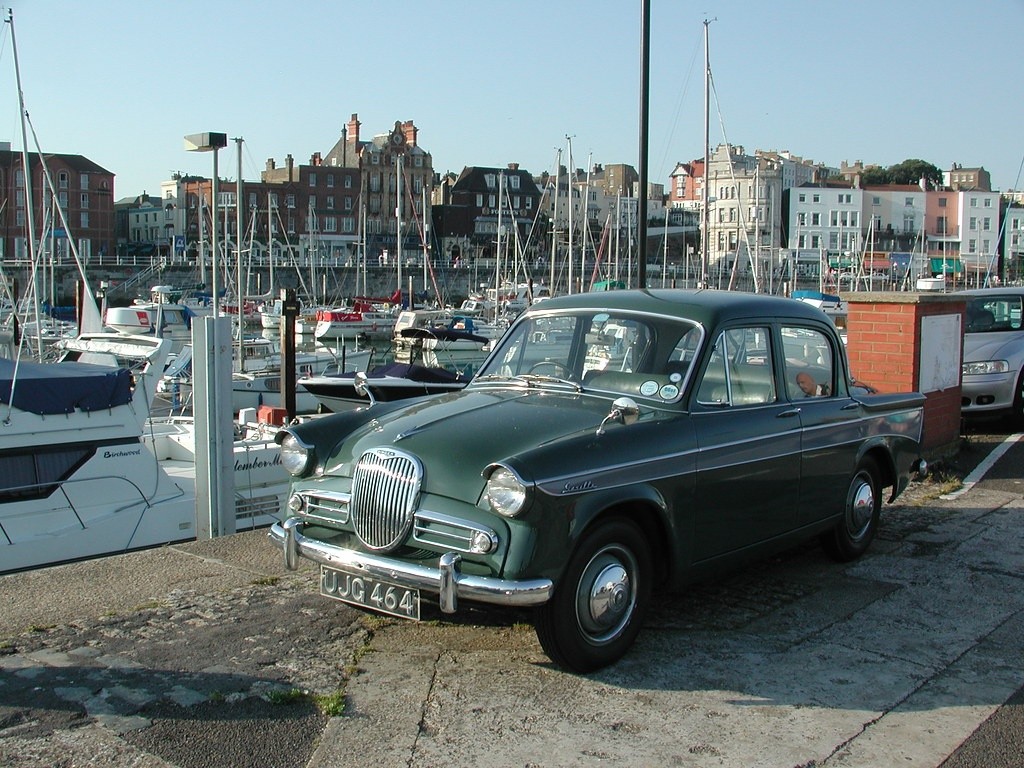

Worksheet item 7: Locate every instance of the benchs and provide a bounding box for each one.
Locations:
[583,358,833,406]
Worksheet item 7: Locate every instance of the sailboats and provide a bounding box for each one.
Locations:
[106,19,961,414]
[1,7,296,573]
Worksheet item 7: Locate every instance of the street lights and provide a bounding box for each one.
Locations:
[185,131,227,538]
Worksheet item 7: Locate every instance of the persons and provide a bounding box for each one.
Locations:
[796,372,831,398]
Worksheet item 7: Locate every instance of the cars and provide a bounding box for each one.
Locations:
[841,271,856,281]
[886,275,902,282]
[265,291,927,677]
[946,287,1024,422]
[861,273,888,282]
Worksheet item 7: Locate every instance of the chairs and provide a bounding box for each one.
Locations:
[971,309,996,328]
